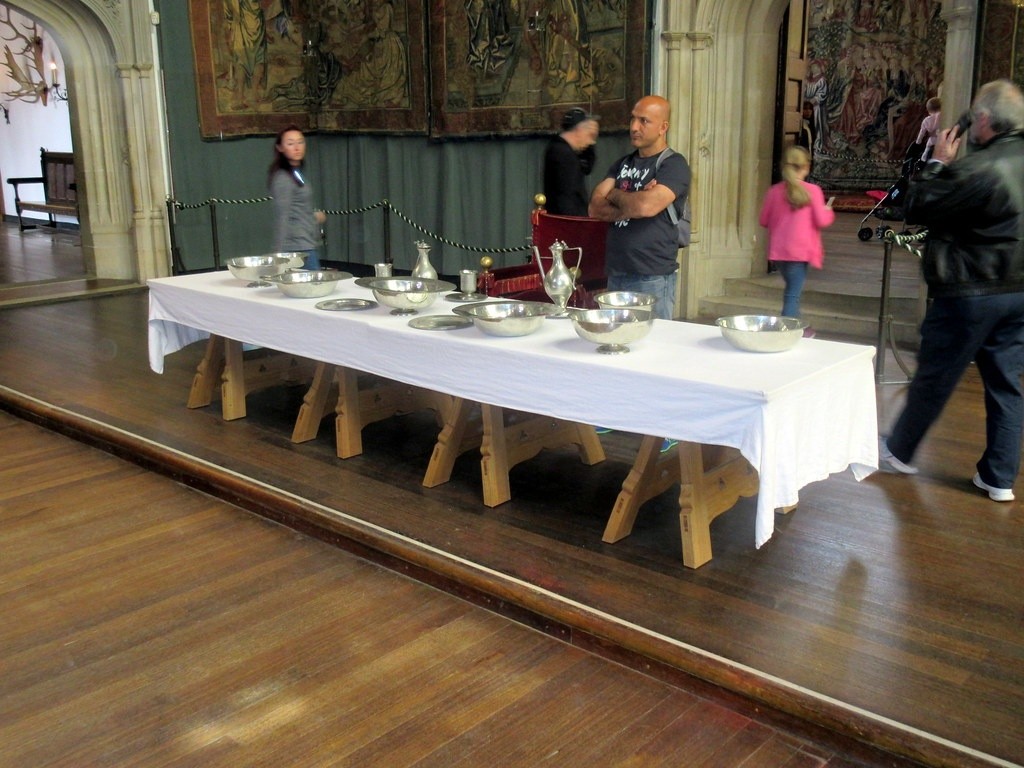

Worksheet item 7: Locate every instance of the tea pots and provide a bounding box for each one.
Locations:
[533,237,582,318]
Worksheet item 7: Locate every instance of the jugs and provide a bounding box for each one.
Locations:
[411,240,439,281]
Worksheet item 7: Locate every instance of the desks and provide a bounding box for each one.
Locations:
[147,270,878,570]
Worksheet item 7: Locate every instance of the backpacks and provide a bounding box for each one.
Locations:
[621,148,693,249]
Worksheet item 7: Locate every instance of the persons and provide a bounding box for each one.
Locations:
[916,96,941,164]
[543,109,596,215]
[798,101,817,156]
[878,78,1024,501]
[266,128,326,268]
[759,145,835,338]
[588,95,692,319]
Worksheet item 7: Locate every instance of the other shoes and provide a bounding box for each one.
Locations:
[802,328,817,339]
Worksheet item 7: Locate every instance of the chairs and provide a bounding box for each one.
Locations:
[6,148,81,238]
[476,194,608,310]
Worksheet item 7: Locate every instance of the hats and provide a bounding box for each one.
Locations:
[560,107,601,131]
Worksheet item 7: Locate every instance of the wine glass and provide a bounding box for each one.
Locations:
[459,269,479,301]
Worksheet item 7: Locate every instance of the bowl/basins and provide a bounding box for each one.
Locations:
[355,276,458,317]
[452,300,564,336]
[593,291,657,316]
[264,252,308,269]
[265,270,352,298]
[224,256,291,287]
[714,314,811,354]
[567,309,656,356]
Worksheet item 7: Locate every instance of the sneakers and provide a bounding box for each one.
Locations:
[659,437,679,452]
[878,437,917,474]
[594,426,614,434]
[973,471,1015,502]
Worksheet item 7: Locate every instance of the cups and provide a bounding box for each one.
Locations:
[374,263,392,278]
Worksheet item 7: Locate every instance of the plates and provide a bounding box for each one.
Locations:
[445,292,488,304]
[315,299,377,313]
[407,314,475,330]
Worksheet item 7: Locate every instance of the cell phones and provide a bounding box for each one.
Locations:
[946,110,974,141]
